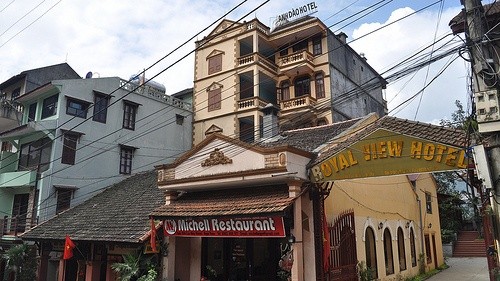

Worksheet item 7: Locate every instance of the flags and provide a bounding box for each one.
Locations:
[150,216,157,253]
[63,234,76,259]
[321,211,332,272]
